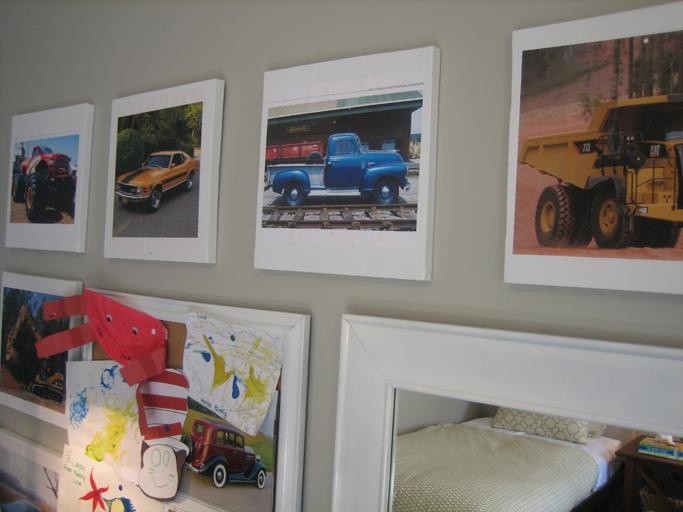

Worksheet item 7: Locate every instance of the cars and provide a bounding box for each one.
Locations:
[116,147,197,210]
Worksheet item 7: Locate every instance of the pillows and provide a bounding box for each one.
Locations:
[489,406,606,446]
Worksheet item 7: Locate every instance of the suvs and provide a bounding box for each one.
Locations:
[13,144,81,223]
[182,418,267,492]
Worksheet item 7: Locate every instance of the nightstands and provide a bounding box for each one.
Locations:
[614,435,683,512]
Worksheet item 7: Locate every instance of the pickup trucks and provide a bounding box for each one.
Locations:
[264,130,410,209]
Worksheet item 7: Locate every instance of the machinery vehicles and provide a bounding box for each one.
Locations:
[519,92,681,248]
[2,303,65,403]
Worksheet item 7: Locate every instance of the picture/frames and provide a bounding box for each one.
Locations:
[330,312,683,512]
[1,428,83,511]
[80,288,312,512]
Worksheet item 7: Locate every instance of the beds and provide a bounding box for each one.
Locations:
[391,402,641,512]
